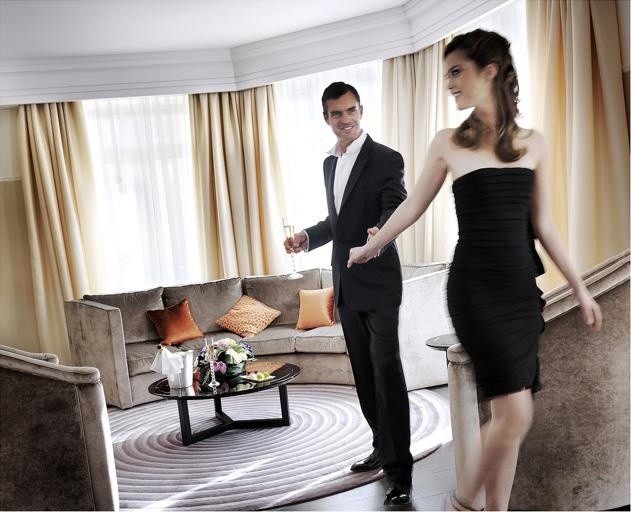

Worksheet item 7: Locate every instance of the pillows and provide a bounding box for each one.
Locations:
[141,295,205,349]
[216,293,285,338]
[292,281,337,333]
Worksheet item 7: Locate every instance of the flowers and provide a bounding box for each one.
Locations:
[192,338,255,386]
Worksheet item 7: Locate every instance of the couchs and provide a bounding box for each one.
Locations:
[61,257,450,408]
[0,345,121,509]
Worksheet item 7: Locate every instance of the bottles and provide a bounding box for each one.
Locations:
[155,344,164,352]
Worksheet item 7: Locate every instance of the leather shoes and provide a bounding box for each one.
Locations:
[351,448,383,472]
[383,481,412,508]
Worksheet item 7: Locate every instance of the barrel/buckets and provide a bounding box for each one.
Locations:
[165,350,195,390]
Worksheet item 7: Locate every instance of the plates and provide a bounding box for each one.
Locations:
[240,372,276,382]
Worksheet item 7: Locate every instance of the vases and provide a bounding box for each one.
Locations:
[214,359,247,379]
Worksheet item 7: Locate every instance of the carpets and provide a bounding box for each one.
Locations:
[105,385,450,509]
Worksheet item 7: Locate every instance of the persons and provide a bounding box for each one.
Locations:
[282,83,415,512]
[348,29,606,512]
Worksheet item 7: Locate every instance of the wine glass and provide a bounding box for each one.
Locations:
[205,337,220,387]
[282,215,305,281]
